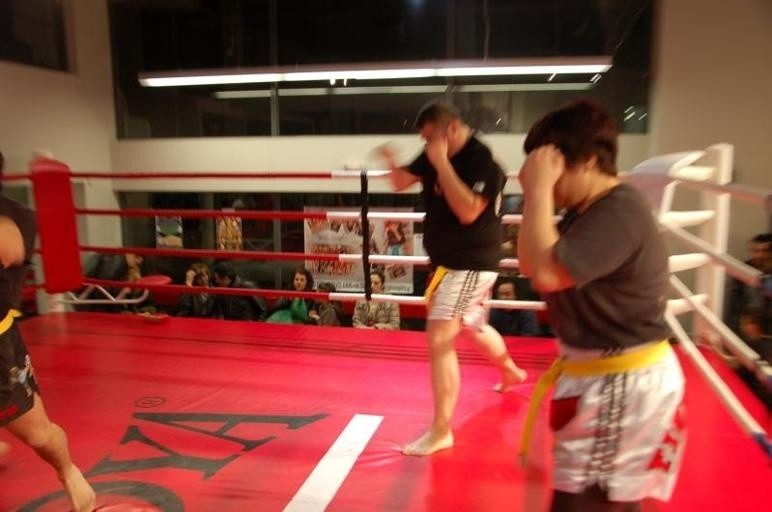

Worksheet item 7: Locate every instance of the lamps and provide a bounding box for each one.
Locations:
[135,4,615,93]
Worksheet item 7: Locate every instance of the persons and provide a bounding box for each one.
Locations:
[0,151,97,512]
[493,282,523,330]
[352,272,401,331]
[517,99,687,512]
[726,232,771,410]
[379,101,528,457]
[175,262,342,326]
